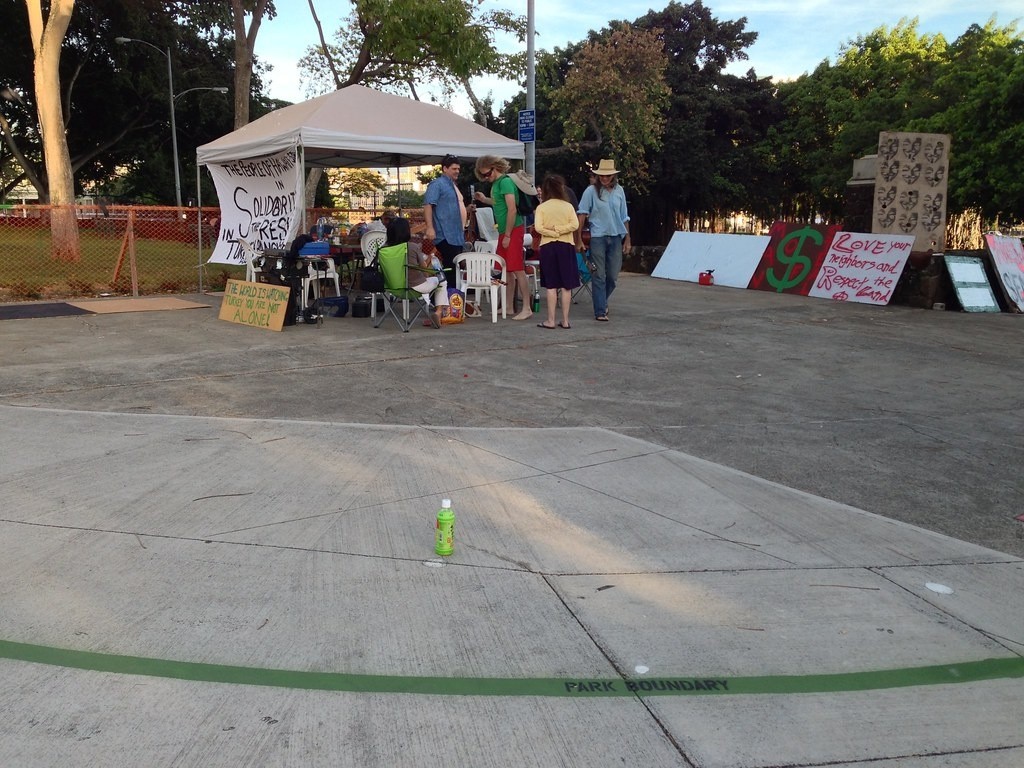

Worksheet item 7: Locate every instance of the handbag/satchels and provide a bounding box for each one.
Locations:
[361,252,384,293]
[440,287,465,323]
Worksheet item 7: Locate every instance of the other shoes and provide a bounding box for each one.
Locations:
[431,314,442,327]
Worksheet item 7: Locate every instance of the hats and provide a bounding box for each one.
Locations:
[507,170,538,196]
[592,159,621,175]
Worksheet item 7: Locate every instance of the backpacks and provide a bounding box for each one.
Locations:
[499,176,540,215]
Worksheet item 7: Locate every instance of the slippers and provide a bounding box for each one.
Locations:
[558,320,571,329]
[597,307,609,321]
[537,321,555,329]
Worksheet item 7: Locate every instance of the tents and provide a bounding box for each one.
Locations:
[194,80,536,296]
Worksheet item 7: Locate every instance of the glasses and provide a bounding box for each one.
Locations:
[481,167,494,177]
[446,155,457,162]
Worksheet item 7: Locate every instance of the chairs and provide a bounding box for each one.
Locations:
[360,229,387,268]
[474,241,495,271]
[570,249,593,304]
[301,257,340,310]
[474,207,499,241]
[453,252,507,324]
[236,237,262,283]
[523,233,540,289]
[373,237,443,332]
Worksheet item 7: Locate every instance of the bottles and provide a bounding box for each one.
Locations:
[530,293,534,312]
[317,215,323,241]
[435,498,455,555]
[430,252,446,282]
[533,291,540,312]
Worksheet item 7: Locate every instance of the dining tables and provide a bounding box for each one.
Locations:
[329,236,365,297]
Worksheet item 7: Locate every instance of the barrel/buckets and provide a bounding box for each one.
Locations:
[699,270,714,285]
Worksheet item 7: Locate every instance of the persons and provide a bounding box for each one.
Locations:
[588,172,598,184]
[377,217,450,327]
[535,184,544,204]
[368,215,388,233]
[473,153,533,320]
[382,211,398,231]
[573,158,631,322]
[533,173,581,329]
